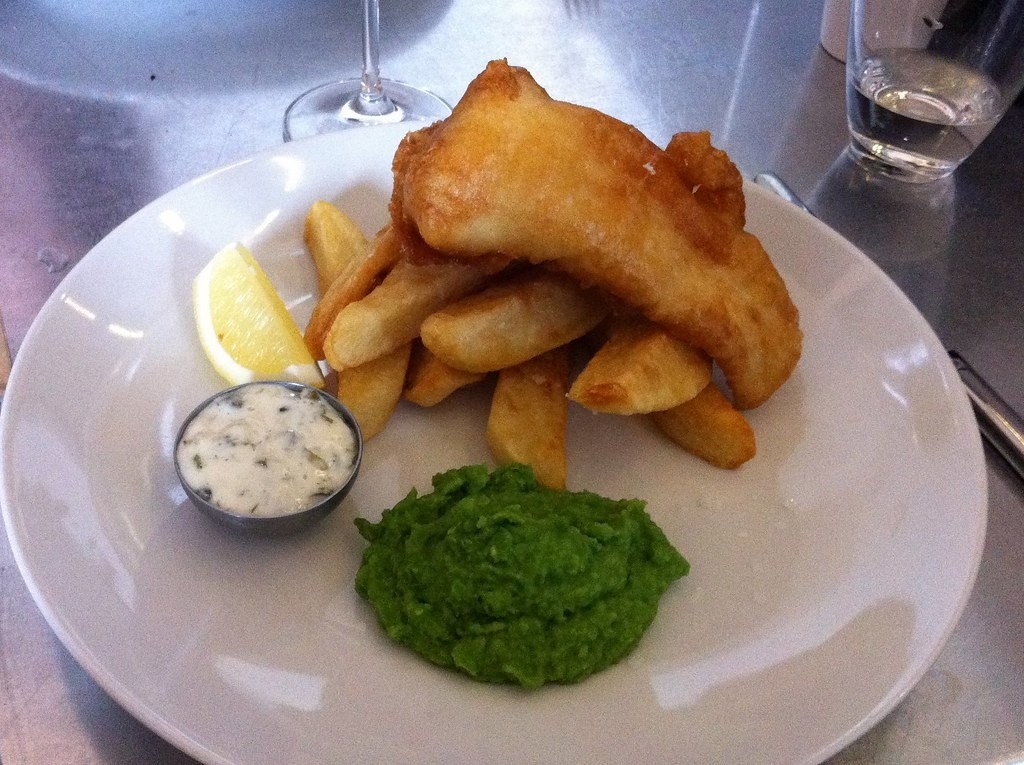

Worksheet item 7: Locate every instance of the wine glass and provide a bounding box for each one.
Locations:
[283,0,454,141]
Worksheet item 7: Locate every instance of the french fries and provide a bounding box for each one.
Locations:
[299,198,756,492]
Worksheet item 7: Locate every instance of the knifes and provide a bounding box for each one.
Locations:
[752,172,1024,481]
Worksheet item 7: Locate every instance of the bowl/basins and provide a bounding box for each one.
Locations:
[173,381,363,536]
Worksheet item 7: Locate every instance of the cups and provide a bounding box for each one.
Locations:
[846,1,1024,184]
[821,1,945,63]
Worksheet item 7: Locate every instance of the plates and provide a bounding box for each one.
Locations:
[0,139,987,764]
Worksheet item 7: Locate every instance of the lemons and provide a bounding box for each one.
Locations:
[193,238,328,393]
